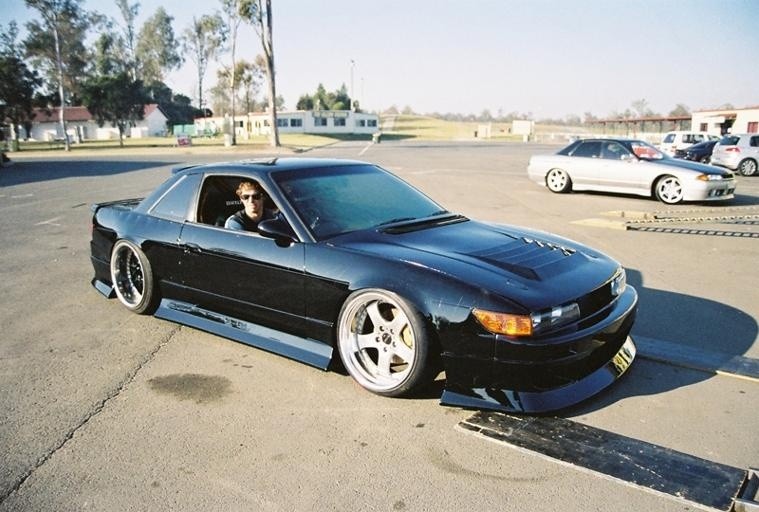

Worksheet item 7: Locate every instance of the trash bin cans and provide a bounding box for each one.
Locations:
[523,135,529,142]
[372,133,382,143]
[7,140,18,152]
[224,133,232,148]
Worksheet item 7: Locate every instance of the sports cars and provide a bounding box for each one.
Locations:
[88,156,638,416]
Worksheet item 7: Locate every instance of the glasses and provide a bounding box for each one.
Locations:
[241,195,262,200]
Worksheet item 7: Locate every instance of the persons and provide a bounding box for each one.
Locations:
[224,178,286,233]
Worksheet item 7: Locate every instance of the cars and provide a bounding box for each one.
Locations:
[527,130,759,205]
